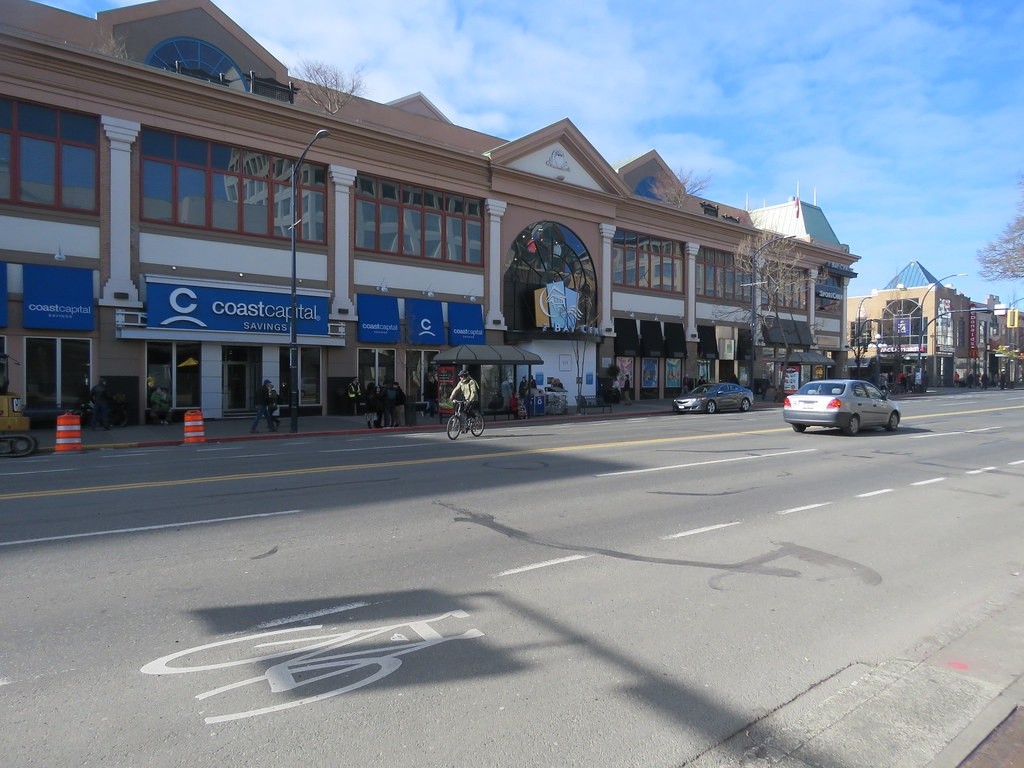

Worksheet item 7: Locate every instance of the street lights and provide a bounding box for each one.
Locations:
[290,130,331,433]
[918,273,969,365]
[750,235,797,388]
[857,296,881,379]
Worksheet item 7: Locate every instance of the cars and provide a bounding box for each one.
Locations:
[783,379,902,436]
[300,376,317,398]
[671,382,754,414]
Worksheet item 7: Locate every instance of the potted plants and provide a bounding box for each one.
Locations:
[608,365,621,383]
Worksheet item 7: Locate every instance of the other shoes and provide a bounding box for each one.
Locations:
[470,418,475,423]
[628,403,632,406]
[624,403,627,405]
[276,420,280,427]
[269,428,278,432]
[460,429,467,434]
[249,429,259,433]
[368,423,401,429]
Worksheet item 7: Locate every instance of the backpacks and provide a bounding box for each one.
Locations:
[399,389,406,405]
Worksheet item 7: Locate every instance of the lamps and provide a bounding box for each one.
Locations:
[0,353,22,364]
[651,313,658,320]
[377,276,389,293]
[707,317,714,324]
[625,310,635,317]
[463,287,478,302]
[422,282,434,297]
[54,242,67,263]
[676,314,683,320]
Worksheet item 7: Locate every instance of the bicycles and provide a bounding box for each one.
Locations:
[70,402,129,429]
[447,400,485,440]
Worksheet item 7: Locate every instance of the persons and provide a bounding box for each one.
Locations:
[879,370,927,393]
[90,377,111,430]
[954,371,1007,390]
[149,386,173,425]
[509,375,537,420]
[623,375,632,405]
[682,373,689,394]
[347,377,407,428]
[698,376,705,385]
[596,371,603,396]
[250,379,279,433]
[422,376,437,417]
[448,370,480,433]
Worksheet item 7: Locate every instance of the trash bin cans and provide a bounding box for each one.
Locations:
[524,389,544,417]
[545,387,569,415]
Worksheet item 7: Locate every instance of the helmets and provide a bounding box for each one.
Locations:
[458,369,469,376]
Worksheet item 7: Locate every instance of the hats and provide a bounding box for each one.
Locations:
[264,380,272,384]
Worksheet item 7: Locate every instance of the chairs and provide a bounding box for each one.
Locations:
[832,388,842,394]
[807,390,817,395]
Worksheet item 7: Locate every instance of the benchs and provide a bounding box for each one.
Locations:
[575,395,612,416]
